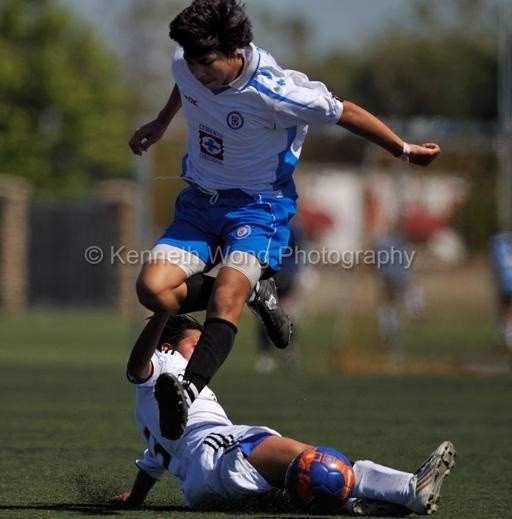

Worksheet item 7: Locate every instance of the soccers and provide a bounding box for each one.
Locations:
[285,447,354,513]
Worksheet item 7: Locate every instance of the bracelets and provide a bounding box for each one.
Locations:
[396,140,410,164]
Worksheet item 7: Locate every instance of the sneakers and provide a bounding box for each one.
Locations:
[417,440,456,514]
[246,278,293,349]
[154,372,188,440]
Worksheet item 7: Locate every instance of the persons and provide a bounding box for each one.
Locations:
[127,1,444,442]
[106,303,458,515]
